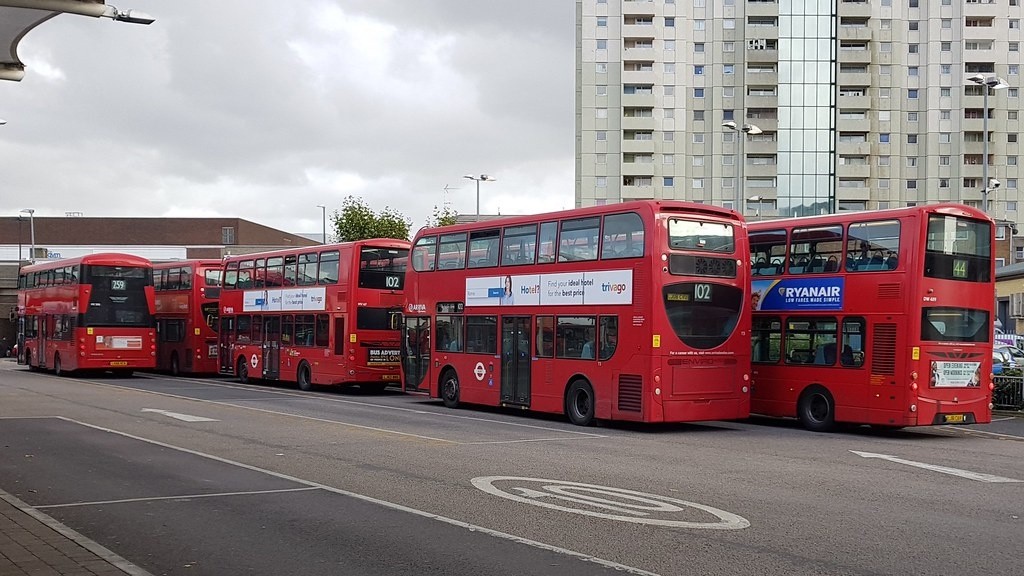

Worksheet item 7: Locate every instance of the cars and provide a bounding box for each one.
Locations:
[992,327,1024,376]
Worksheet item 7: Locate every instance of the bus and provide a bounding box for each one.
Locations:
[399,198,753,429]
[333,229,644,339]
[747,200,996,433]
[152,259,260,379]
[216,234,430,392]
[11,252,158,380]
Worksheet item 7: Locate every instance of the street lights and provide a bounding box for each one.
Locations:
[965,74,1009,214]
[19,208,35,263]
[316,203,326,245]
[721,122,765,218]
[463,174,495,223]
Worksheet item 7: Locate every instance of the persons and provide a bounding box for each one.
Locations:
[500,276,514,305]
[261,290,269,311]
[751,292,761,310]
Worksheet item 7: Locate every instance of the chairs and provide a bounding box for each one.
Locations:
[429,247,641,271]
[244,276,253,289]
[233,278,244,289]
[752,251,896,274]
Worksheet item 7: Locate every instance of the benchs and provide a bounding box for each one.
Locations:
[812,343,854,366]
